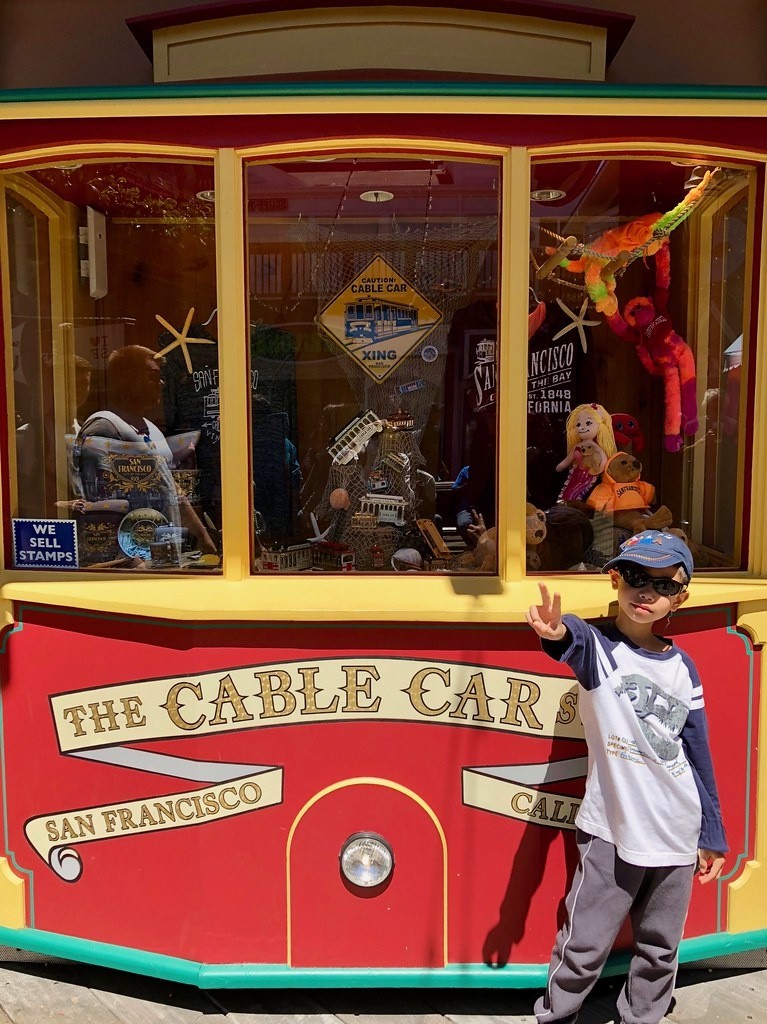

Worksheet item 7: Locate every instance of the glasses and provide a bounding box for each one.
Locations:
[617,565,683,597]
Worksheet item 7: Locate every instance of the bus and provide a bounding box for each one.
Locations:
[326,409,385,466]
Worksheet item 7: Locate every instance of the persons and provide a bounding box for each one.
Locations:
[197,393,307,548]
[526,530,727,1024]
[70,343,221,574]
[17,357,91,518]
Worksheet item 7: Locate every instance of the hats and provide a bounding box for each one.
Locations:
[601,530,694,579]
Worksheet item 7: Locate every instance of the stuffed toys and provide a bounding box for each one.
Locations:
[456,502,546,574]
[582,211,699,452]
[588,451,675,530]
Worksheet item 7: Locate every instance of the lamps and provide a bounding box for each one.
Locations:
[339,832,393,887]
